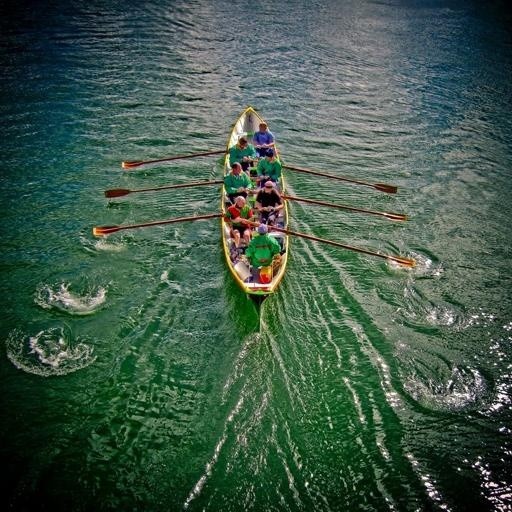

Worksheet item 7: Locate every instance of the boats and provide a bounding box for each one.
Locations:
[221,105,289,295]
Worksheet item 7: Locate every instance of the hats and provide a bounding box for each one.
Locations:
[265,181,273,187]
[258,224,268,234]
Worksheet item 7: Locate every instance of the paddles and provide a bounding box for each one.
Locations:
[93,213,225,237]
[122,151,226,169]
[249,191,408,223]
[105,180,223,198]
[253,160,397,193]
[249,221,415,267]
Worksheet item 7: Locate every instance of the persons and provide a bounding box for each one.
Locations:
[223,195,256,256]
[256,146,282,194]
[222,161,253,208]
[245,221,283,277]
[228,135,256,179]
[254,180,284,232]
[252,121,277,162]
